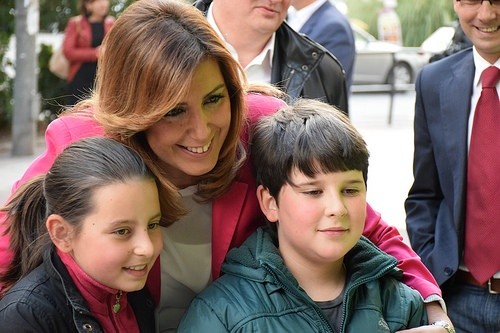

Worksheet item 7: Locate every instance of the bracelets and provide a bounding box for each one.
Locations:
[431,321,456,333]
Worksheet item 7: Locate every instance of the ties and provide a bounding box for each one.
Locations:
[461,65,500,285]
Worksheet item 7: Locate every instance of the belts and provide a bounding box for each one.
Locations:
[456,267,500,294]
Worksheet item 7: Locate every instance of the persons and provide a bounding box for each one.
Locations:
[404,0,500,333]
[290,0,356,104]
[189,0,349,122]
[177,99,429,332]
[0,138,164,333]
[62,0,117,105]
[376,0,403,48]
[0,0,456,332]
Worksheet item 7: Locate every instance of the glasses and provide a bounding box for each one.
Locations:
[455,0,500,8]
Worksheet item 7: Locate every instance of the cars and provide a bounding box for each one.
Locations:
[421,26,456,55]
[350,23,428,85]
